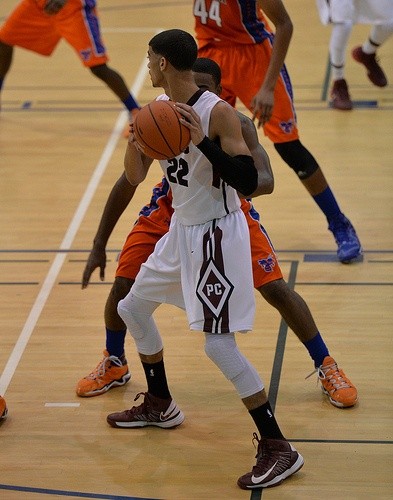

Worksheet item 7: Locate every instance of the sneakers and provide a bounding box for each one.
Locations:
[327,214,362,263]
[125,105,143,137]
[106,392,185,429]
[352,46,389,88]
[330,78,353,110]
[237,432,304,489]
[75,349,130,397]
[319,355,360,408]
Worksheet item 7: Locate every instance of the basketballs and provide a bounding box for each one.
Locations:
[133,100,191,160]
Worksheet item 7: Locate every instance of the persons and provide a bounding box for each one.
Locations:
[0,395,8,427]
[190,0,363,266]
[0,0,142,137]
[105,28,304,490]
[76,57,358,409]
[317,0,393,110]
